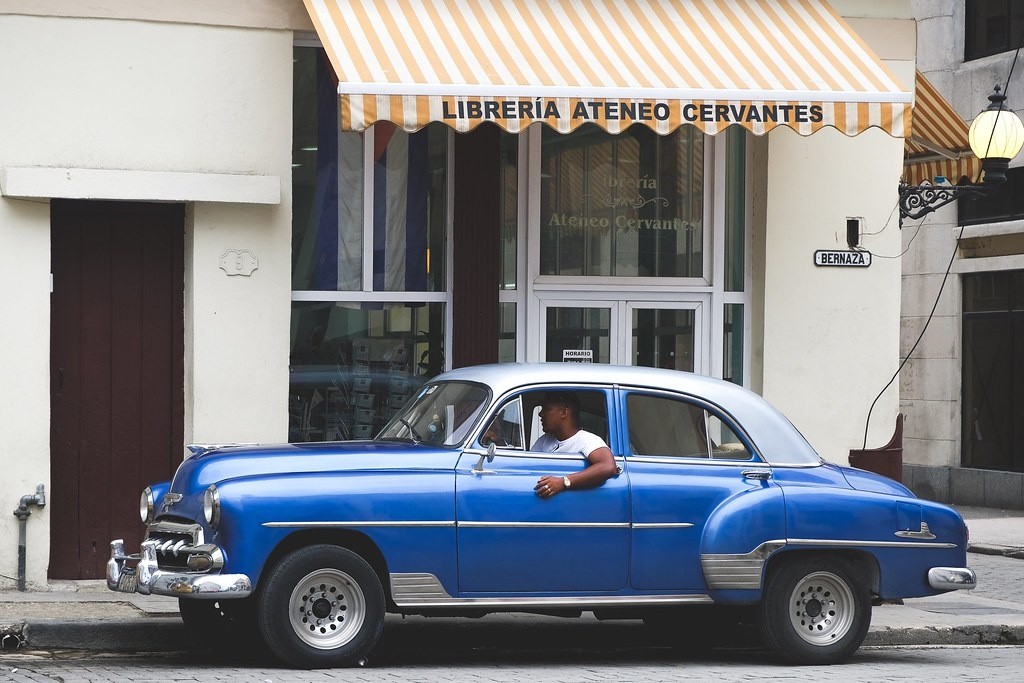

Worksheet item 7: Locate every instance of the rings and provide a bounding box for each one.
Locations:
[545,484,551,493]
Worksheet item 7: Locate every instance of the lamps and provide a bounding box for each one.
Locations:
[897,84,1024,230]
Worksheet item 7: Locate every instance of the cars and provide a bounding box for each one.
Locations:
[107,363,979,669]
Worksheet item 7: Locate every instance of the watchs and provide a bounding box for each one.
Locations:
[563,476,570,489]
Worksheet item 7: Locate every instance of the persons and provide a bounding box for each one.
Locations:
[532,388,618,499]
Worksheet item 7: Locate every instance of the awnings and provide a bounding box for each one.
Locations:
[299,0,912,138]
[905,69,999,188]
